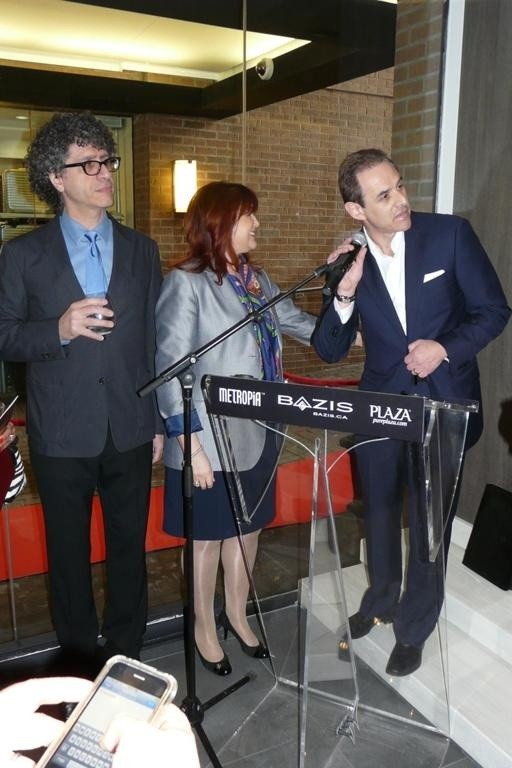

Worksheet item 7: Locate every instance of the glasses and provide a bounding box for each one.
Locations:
[60,157,120,176]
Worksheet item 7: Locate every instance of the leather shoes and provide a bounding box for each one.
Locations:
[349,609,394,640]
[385,642,423,676]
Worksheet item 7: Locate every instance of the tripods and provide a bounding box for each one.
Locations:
[137,265,320,768]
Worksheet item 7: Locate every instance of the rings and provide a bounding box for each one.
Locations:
[414,369,419,375]
[193,480,199,485]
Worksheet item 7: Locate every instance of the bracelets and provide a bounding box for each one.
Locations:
[191,446,202,458]
[335,290,356,303]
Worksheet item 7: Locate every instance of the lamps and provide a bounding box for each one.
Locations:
[172,159,198,214]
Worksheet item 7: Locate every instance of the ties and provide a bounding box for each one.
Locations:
[84,232,106,301]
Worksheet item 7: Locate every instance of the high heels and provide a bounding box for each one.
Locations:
[189,624,232,677]
[219,607,270,658]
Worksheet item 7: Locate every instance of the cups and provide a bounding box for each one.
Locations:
[84,293,116,336]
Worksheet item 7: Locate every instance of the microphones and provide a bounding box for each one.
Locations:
[321,229,367,295]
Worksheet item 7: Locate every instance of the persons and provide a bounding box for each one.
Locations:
[311,150,511,676]
[0,676,202,768]
[0,111,168,681]
[155,183,364,674]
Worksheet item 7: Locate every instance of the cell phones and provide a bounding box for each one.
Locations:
[36,653,176,768]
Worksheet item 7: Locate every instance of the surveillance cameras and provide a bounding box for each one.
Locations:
[256,57,274,81]
